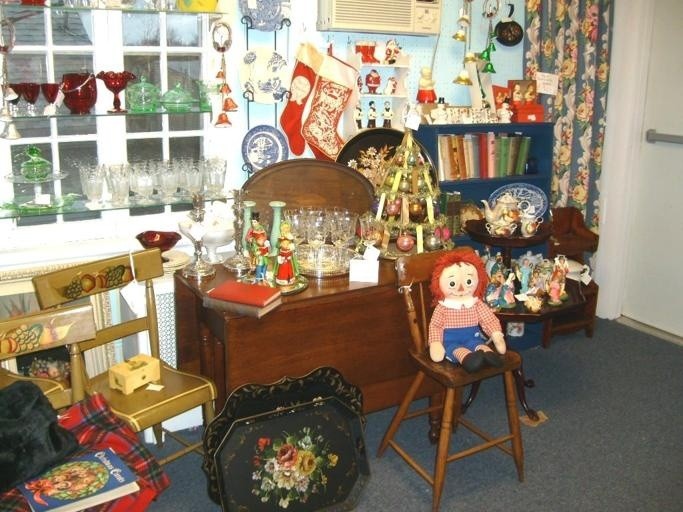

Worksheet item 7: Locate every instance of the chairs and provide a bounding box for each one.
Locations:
[0,302,169,511]
[31,246,218,467]
[379,245,525,512]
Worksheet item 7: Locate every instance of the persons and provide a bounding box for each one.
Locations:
[244,212,297,290]
[421,247,508,371]
[486,253,568,315]
[350,39,540,129]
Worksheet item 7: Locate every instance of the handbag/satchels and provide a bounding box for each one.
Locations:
[0,380,79,491]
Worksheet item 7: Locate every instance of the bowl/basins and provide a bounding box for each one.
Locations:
[158,81,200,113]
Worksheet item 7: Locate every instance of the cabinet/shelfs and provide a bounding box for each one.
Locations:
[342,42,410,137]
[462,208,598,348]
[2,2,234,221]
[419,122,551,351]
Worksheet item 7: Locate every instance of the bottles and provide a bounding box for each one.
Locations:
[520,214,537,237]
[266,200,286,249]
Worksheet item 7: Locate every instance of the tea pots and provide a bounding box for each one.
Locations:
[478,192,521,238]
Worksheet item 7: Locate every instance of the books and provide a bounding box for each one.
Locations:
[18,446,141,511]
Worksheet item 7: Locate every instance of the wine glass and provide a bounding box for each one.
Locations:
[1,81,59,118]
[94,70,137,114]
[283,204,378,273]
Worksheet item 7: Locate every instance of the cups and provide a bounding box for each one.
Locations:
[76,156,228,208]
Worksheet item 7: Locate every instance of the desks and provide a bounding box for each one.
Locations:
[174,259,446,446]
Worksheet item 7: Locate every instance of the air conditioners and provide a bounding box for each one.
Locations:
[317,0,440,38]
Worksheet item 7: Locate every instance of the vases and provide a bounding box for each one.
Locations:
[268,201,287,254]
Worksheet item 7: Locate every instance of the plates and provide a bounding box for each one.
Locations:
[488,182,549,224]
[241,124,290,174]
[237,0,290,33]
[237,48,291,105]
